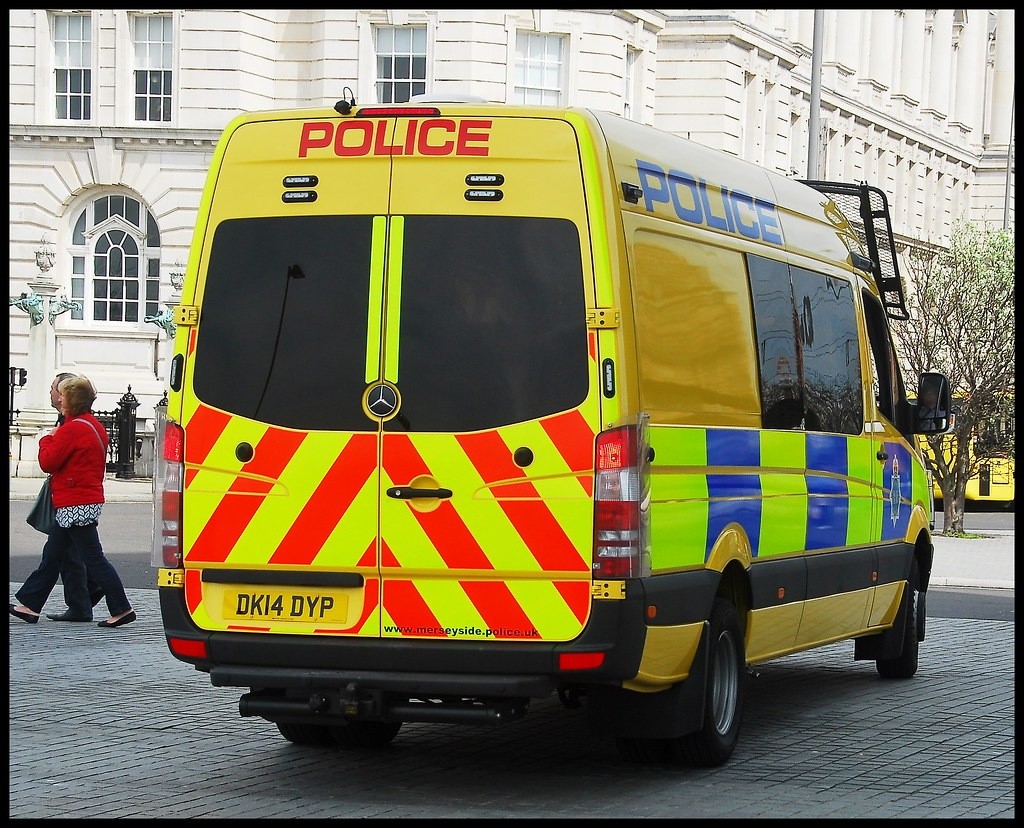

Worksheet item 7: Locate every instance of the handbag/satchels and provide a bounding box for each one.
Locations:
[26,474,57,536]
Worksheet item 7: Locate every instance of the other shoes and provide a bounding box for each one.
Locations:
[9,603,39,624]
[46,609,94,624]
[97,611,137,628]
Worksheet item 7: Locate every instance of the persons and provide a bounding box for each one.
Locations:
[919,387,946,431]
[9,372,136,629]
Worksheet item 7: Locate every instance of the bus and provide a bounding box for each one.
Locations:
[903,383,1017,512]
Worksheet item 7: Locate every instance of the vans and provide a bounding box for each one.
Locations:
[156,86,954,776]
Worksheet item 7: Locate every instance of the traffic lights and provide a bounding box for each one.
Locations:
[19,368,28,387]
